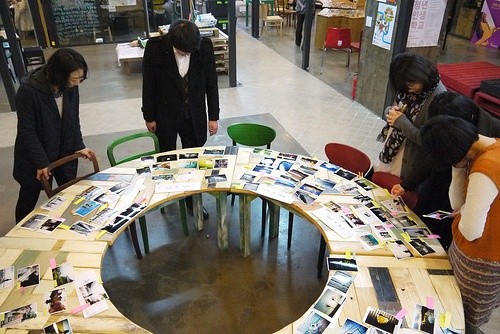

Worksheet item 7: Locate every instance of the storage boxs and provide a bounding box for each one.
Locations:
[23,46,45,72]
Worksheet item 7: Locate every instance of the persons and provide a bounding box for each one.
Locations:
[292,0,315,51]
[17,265,39,288]
[375,53,447,178]
[13,49,95,225]
[391,91,500,334]
[141,20,220,218]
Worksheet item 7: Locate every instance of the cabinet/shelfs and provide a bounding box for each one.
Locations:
[112,13,136,33]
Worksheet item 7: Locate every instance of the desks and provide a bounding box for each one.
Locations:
[314,0,365,49]
[435,60,500,118]
[0,146,466,334]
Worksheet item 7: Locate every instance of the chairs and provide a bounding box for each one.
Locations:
[348,28,363,68]
[261,4,283,38]
[290,143,420,281]
[277,0,297,28]
[108,132,188,255]
[39,152,143,259]
[227,124,277,206]
[320,27,351,75]
